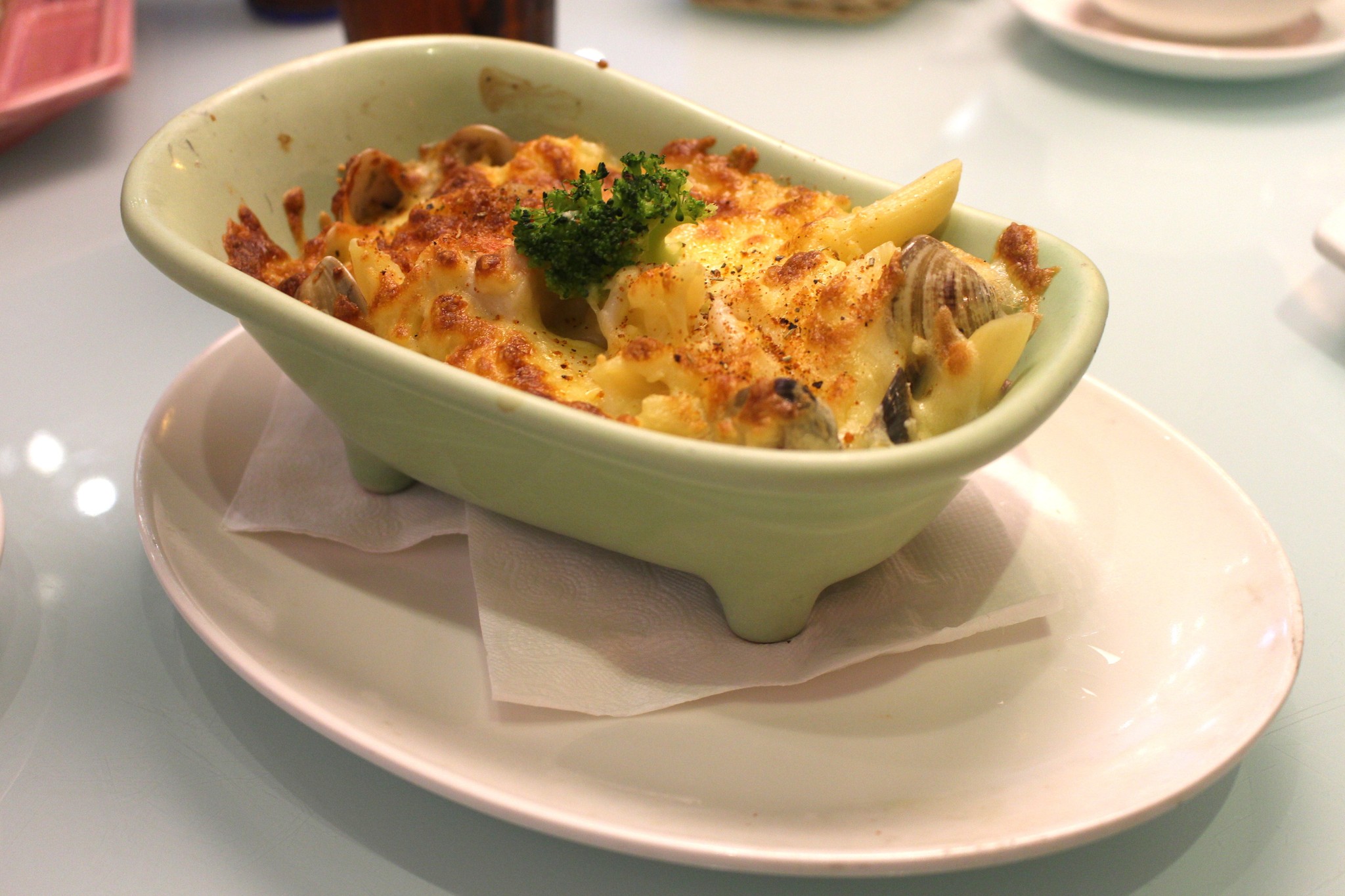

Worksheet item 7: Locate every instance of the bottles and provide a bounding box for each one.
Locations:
[339,0,556,52]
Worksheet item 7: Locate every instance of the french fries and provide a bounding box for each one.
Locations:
[967,313,1034,403]
[812,157,961,256]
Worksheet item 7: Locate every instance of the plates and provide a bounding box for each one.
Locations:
[1016,0,1345,78]
[135,328,1306,877]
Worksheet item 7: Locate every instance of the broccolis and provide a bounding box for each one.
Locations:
[509,147,718,303]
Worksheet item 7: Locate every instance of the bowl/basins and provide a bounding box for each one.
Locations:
[122,33,1107,643]
